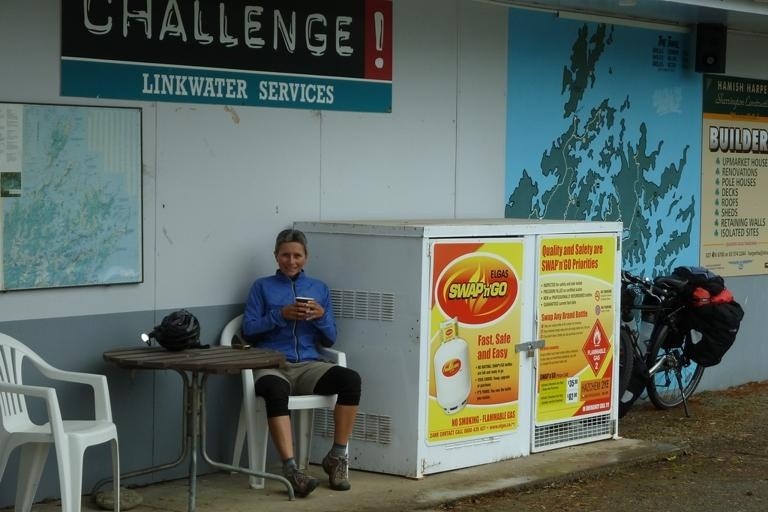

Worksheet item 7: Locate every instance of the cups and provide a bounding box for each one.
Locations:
[294,295,315,305]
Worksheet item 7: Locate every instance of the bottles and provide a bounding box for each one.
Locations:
[432,316,472,414]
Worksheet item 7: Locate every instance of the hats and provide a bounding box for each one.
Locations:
[619,282,641,322]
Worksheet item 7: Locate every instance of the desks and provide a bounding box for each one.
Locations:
[99,342,286,512]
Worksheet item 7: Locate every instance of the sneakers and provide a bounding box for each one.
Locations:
[287,465,320,499]
[321,451,353,492]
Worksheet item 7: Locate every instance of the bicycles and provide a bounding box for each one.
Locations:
[619,269,706,419]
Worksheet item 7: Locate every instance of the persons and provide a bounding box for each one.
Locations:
[241,228,363,496]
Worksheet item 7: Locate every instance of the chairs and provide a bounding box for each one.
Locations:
[219,310,352,491]
[0,330,123,512]
